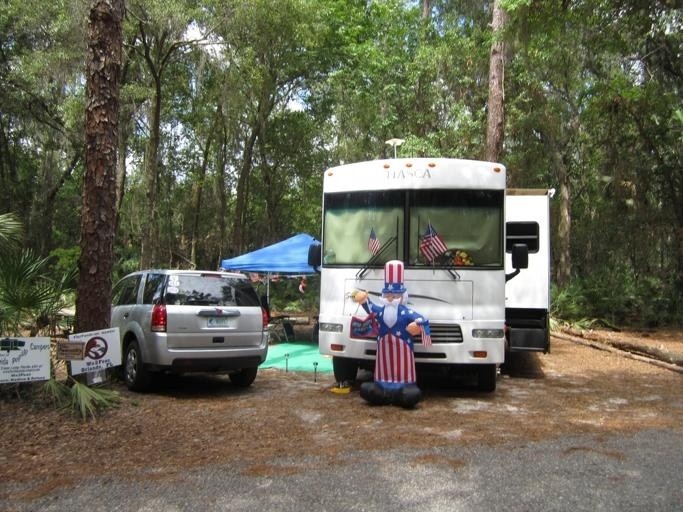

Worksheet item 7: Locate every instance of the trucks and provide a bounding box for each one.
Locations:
[317,157,550,392]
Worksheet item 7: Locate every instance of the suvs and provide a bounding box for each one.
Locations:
[104,269,268,388]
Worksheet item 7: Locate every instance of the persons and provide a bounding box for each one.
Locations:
[351,260,432,410]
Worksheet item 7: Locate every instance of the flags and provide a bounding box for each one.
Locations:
[368,227,380,256]
[420,223,447,263]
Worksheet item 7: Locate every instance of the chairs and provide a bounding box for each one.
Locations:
[260,295,291,342]
